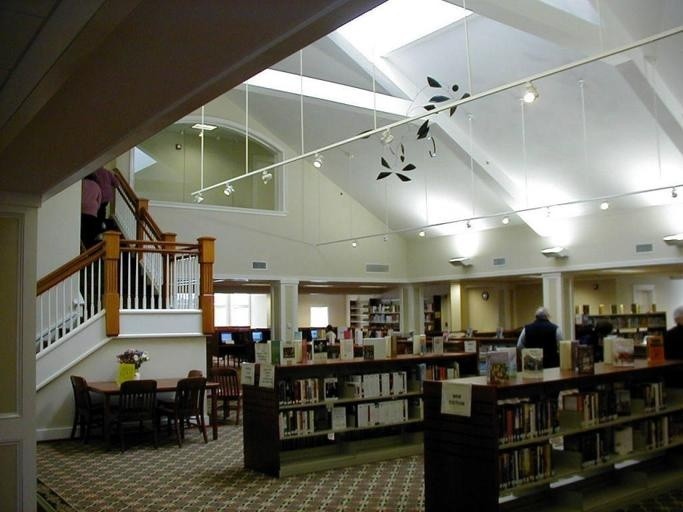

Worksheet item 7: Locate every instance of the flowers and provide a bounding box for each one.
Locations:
[117,347,150,369]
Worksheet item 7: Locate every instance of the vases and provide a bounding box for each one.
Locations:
[118,362,138,383]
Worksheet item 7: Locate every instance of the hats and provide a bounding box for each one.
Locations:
[535,308,551,317]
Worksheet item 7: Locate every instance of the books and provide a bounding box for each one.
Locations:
[255,335,476,439]
[587,314,664,347]
[480,336,665,385]
[371,303,435,331]
[497,381,683,491]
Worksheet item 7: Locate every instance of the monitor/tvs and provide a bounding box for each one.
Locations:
[252,331,264,343]
[311,329,319,339]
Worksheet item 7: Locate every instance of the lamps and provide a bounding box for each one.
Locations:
[311,60,682,251]
[192,124,217,131]
[189,1,679,205]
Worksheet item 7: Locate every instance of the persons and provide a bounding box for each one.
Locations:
[326,325,336,344]
[576,317,613,362]
[81,175,102,238]
[344,327,352,339]
[517,306,563,368]
[375,327,382,338]
[664,306,683,360]
[94,167,118,222]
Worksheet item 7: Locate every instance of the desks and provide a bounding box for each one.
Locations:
[88,379,221,403]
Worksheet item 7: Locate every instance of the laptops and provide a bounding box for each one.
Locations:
[220,331,232,344]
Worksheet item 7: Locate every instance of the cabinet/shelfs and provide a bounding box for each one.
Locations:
[590,314,667,359]
[419,355,683,512]
[349,299,401,338]
[240,348,483,477]
[326,296,397,331]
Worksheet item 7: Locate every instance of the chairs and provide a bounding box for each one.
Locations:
[103,379,160,452]
[210,368,242,424]
[68,375,118,447]
[158,377,209,448]
